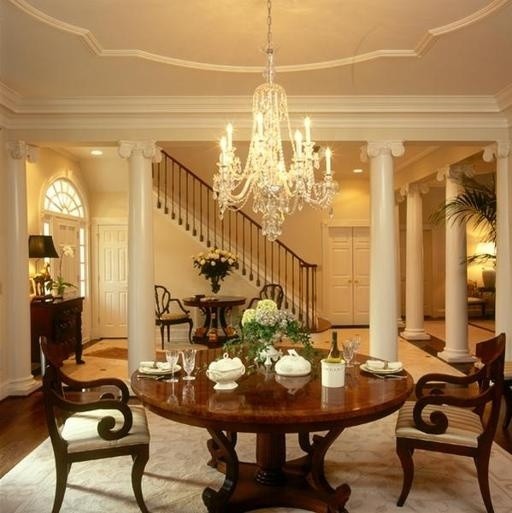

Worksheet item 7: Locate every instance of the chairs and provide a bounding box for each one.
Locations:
[36,334,153,513]
[466,279,489,318]
[393,332,506,511]
[238,284,284,343]
[154,283,193,351]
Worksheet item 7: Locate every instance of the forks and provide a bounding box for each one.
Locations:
[136,373,165,380]
[373,373,408,379]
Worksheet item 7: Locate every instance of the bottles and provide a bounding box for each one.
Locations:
[326,330,341,362]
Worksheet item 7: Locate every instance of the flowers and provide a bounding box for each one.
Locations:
[44,242,78,289]
[219,300,317,339]
[191,248,238,286]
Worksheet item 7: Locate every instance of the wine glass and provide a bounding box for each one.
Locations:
[342,340,354,367]
[351,333,361,364]
[164,348,179,382]
[180,346,196,380]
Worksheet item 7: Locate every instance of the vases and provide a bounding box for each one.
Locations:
[247,330,285,370]
[205,281,224,298]
[51,286,64,298]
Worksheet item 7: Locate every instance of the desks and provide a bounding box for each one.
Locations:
[476,285,496,317]
[182,293,246,349]
[130,345,415,513]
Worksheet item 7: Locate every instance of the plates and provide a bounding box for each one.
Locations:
[137,361,182,374]
[360,363,404,373]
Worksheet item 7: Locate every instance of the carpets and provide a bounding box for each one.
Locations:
[0,401,512,513]
[54,323,469,388]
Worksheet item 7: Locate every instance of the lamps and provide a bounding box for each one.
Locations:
[27,234,59,298]
[475,241,496,288]
[209,2,341,244]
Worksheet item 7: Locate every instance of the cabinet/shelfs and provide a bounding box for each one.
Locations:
[30,295,87,375]
